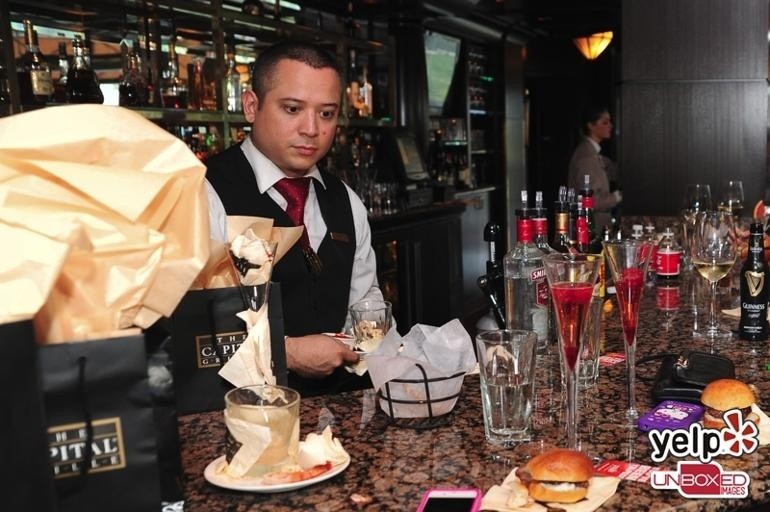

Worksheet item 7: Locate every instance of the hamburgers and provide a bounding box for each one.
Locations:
[515,449,593,504]
[700,378,760,430]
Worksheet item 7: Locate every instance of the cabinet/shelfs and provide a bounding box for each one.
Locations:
[0,1,531,397]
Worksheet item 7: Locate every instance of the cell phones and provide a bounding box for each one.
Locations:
[416,487,483,512]
[637,399,704,433]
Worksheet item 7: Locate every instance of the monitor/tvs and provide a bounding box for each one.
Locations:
[424,30,464,116]
[382,129,432,181]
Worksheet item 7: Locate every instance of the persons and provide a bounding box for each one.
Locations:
[569,107,624,237]
[202,36,396,396]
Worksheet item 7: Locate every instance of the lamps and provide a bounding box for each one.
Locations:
[572,28,614,64]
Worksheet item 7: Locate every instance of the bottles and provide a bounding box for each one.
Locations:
[7,12,399,217]
[502,171,769,361]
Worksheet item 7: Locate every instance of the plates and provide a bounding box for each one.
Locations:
[204,440,352,497]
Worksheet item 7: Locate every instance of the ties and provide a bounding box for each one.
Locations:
[272,177,311,250]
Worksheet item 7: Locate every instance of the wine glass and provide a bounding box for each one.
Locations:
[542,240,654,465]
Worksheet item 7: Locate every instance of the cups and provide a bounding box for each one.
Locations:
[473,329,539,443]
[223,386,302,473]
[343,299,391,347]
